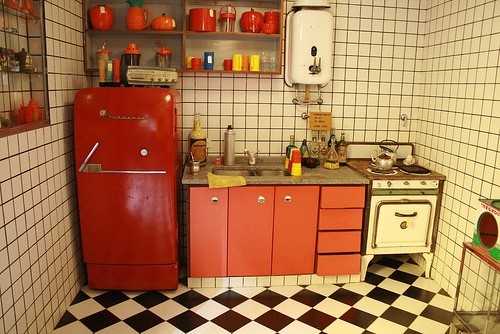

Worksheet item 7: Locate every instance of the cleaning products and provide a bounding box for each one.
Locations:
[284,134,297,169]
[224,125,235,166]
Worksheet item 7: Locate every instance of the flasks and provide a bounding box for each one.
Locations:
[224,124,235,166]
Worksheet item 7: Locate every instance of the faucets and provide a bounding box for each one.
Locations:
[243,149,260,165]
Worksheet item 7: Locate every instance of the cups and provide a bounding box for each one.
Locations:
[224,54,279,71]
[219,4,236,33]
[187,56,201,71]
[0,46,33,72]
[106,57,120,82]
[204,51,214,70]
[190,160,200,175]
[125,6,151,32]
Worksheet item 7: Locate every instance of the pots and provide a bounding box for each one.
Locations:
[240,8,263,33]
[189,7,217,32]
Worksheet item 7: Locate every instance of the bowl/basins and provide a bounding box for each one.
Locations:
[152,13,175,31]
[260,10,280,34]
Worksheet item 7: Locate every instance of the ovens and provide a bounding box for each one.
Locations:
[365,190,439,255]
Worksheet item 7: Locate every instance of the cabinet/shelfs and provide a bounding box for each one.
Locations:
[446,248,500,334]
[188,183,367,276]
[82,0,283,75]
[0,0,50,137]
[364,178,443,279]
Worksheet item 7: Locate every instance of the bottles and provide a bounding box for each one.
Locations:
[300,128,347,171]
[155,46,171,67]
[90,3,115,31]
[288,135,295,145]
[187,112,208,167]
[119,43,144,87]
[96,42,112,82]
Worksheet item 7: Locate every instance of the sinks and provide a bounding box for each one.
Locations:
[254,170,292,176]
[211,169,255,176]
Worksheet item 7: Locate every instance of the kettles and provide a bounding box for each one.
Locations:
[369,138,399,170]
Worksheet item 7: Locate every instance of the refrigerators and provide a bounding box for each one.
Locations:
[73,87,183,290]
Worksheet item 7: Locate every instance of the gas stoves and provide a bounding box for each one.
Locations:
[344,140,446,189]
